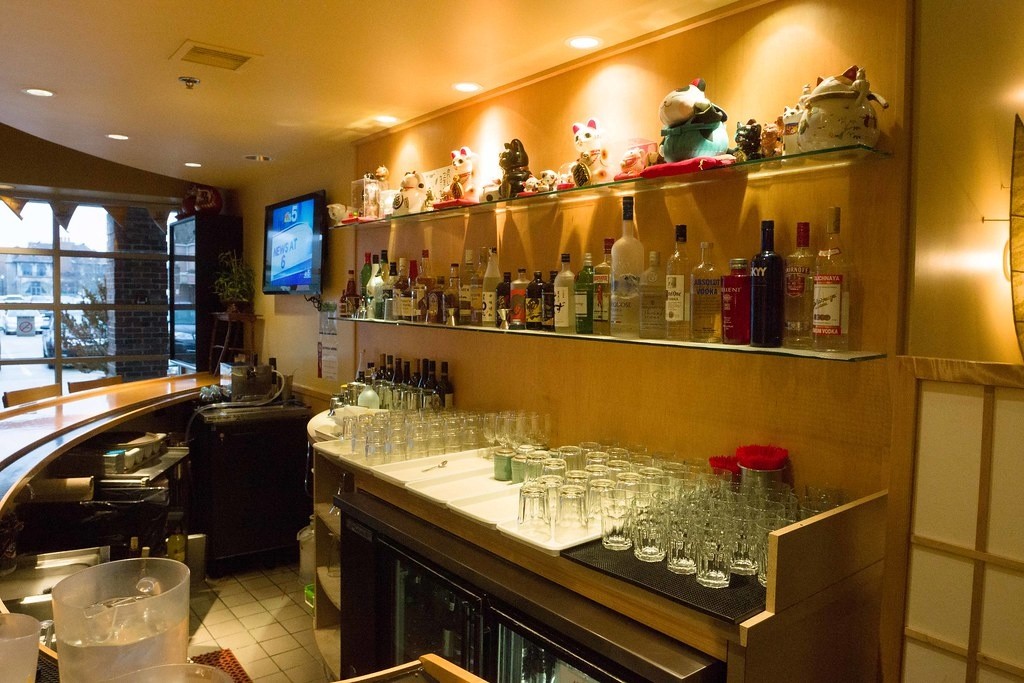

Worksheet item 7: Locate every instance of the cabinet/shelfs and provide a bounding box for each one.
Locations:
[308,441,728,683]
[323,145,891,362]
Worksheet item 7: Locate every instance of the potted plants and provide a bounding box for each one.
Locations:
[212,250,254,314]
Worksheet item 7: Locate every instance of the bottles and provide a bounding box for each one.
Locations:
[297,520,315,585]
[329,353,454,410]
[338,196,851,353]
[127,536,150,558]
[167,521,186,565]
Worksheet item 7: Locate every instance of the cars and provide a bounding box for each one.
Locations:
[40,313,109,370]
[0,295,80,334]
[167,301,197,358]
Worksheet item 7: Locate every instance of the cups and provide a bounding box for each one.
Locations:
[341,407,850,588]
[280,375,293,400]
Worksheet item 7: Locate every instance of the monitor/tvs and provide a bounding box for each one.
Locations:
[261,189,326,295]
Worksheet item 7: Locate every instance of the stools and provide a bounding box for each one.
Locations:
[3,375,122,407]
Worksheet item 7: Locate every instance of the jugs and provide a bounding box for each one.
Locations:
[52,558,190,683]
[103,663,234,683]
[0,613,41,683]
